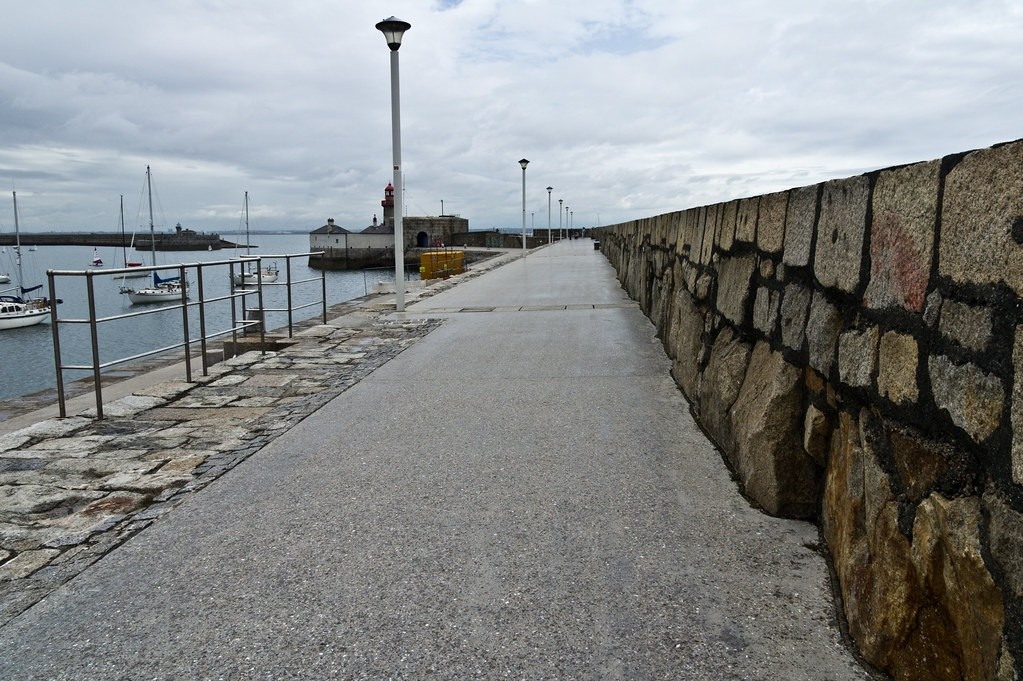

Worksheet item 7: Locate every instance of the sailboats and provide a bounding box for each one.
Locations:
[228,191,279,285]
[28,245,37,251]
[88,247,102,267]
[121,165,191,304]
[109,192,152,279]
[0,191,50,330]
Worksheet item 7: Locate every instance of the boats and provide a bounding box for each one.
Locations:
[0,274,11,283]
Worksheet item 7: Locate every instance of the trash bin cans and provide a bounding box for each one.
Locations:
[594,243,600,250]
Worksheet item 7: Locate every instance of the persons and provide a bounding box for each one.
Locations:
[581,227,586,239]
[569,229,573,240]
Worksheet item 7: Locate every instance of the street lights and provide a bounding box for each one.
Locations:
[546,186,553,244]
[376,14,412,312]
[558,199,563,240]
[570,212,573,228]
[519,158,529,249]
[565,206,569,238]
